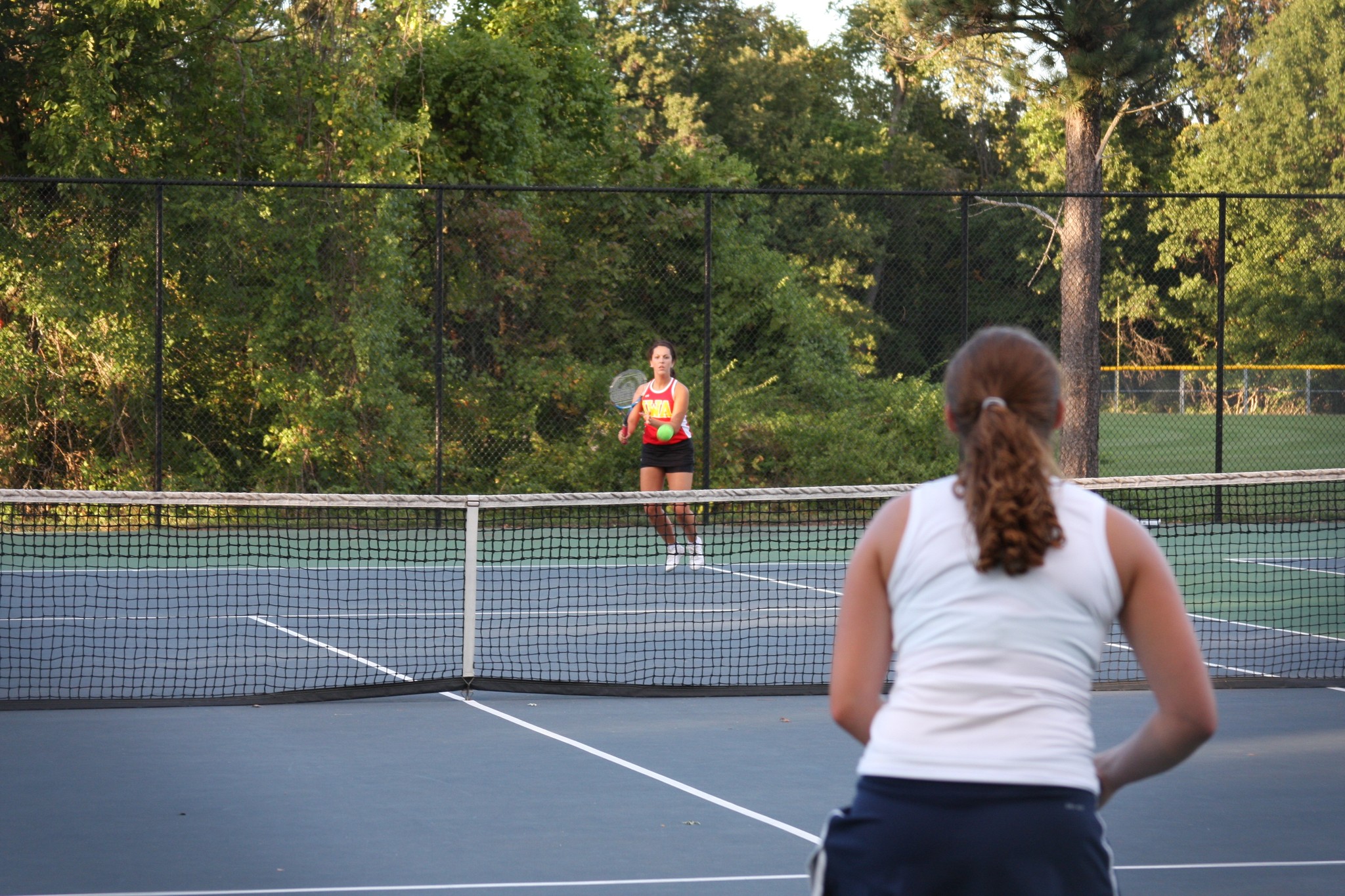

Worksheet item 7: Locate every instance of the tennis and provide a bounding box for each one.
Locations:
[656,423,674,442]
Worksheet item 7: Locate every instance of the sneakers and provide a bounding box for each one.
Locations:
[688,536,705,570]
[665,542,686,571]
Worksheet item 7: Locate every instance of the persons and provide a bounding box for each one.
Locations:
[805,326,1218,896]
[619,340,706,575]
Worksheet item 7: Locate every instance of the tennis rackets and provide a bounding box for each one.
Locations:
[609,369,648,445]
